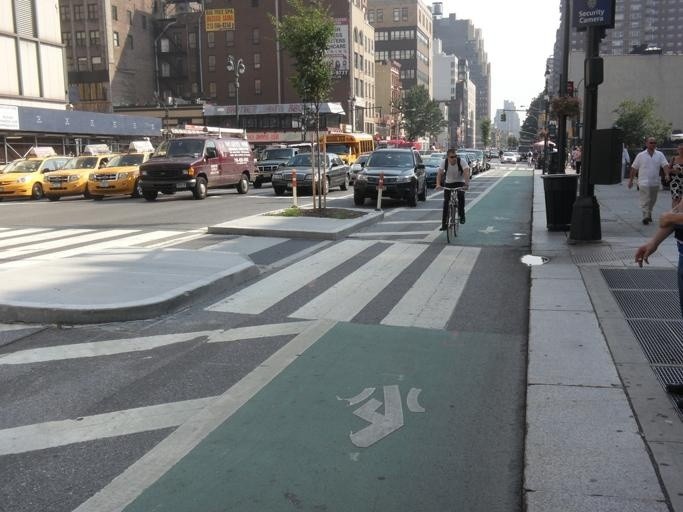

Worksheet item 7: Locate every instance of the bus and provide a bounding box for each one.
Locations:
[318,131,375,166]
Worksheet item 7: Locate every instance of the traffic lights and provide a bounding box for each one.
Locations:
[500,114,507,121]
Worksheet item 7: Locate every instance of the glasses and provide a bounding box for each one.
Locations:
[451,155,457,158]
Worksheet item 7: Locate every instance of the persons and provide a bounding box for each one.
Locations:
[669,144,683,208]
[431,143,435,150]
[436,148,470,230]
[634,193,683,323]
[628,137,670,225]
[568,146,581,174]
[526,150,533,167]
[533,149,538,162]
[621,143,630,184]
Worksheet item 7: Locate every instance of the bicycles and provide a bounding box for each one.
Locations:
[432,184,468,243]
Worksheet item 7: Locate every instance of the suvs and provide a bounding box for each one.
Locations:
[138,123,260,201]
[254,145,302,189]
[351,147,427,208]
[292,142,319,154]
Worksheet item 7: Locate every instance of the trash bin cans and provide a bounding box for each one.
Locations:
[540,174,582,232]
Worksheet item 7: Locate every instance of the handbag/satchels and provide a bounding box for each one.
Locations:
[661,157,675,187]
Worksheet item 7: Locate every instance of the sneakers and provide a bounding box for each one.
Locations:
[460,216,465,223]
[440,225,447,230]
[643,215,652,224]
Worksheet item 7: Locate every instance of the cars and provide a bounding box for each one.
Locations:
[420,147,534,190]
[347,153,371,186]
[85,139,156,201]
[271,151,353,196]
[41,143,123,201]
[669,127,682,143]
[0,144,76,201]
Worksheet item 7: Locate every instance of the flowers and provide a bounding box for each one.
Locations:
[550,96,582,116]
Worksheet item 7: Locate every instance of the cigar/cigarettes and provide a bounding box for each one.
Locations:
[629,257,642,264]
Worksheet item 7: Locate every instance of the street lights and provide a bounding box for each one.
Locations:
[543,62,551,170]
[224,55,246,128]
[393,107,416,148]
